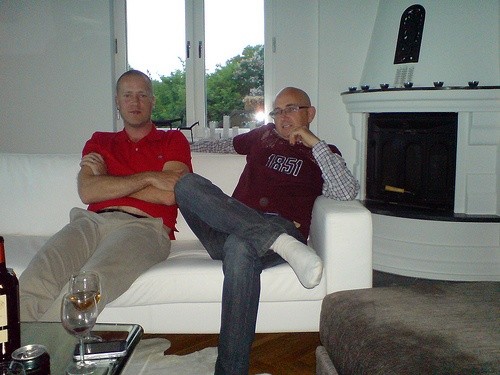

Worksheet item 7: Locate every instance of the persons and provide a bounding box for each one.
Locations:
[174,88,360,375]
[20,71,193,324]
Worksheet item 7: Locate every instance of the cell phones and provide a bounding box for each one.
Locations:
[88,331,128,342]
[73,341,128,361]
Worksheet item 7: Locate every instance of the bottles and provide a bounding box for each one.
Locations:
[0,236,21,364]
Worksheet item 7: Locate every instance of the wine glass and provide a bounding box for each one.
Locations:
[68,270,103,346]
[60,290,97,375]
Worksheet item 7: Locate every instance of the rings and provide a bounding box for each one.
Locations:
[91,159,95,163]
[97,159,101,162]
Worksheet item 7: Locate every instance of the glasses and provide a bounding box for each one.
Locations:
[269,106,309,117]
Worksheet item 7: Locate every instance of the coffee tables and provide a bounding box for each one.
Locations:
[21,321,145,375]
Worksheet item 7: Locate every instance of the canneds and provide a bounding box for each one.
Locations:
[8,344,50,375]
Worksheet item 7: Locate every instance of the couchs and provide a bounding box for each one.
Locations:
[0,153,373,334]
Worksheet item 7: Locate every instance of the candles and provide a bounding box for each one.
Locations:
[232,125,238,140]
[210,120,216,141]
[223,113,230,139]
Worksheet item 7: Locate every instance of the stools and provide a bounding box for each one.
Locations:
[315,282,500,375]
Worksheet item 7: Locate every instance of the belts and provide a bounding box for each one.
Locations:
[96,209,148,218]
